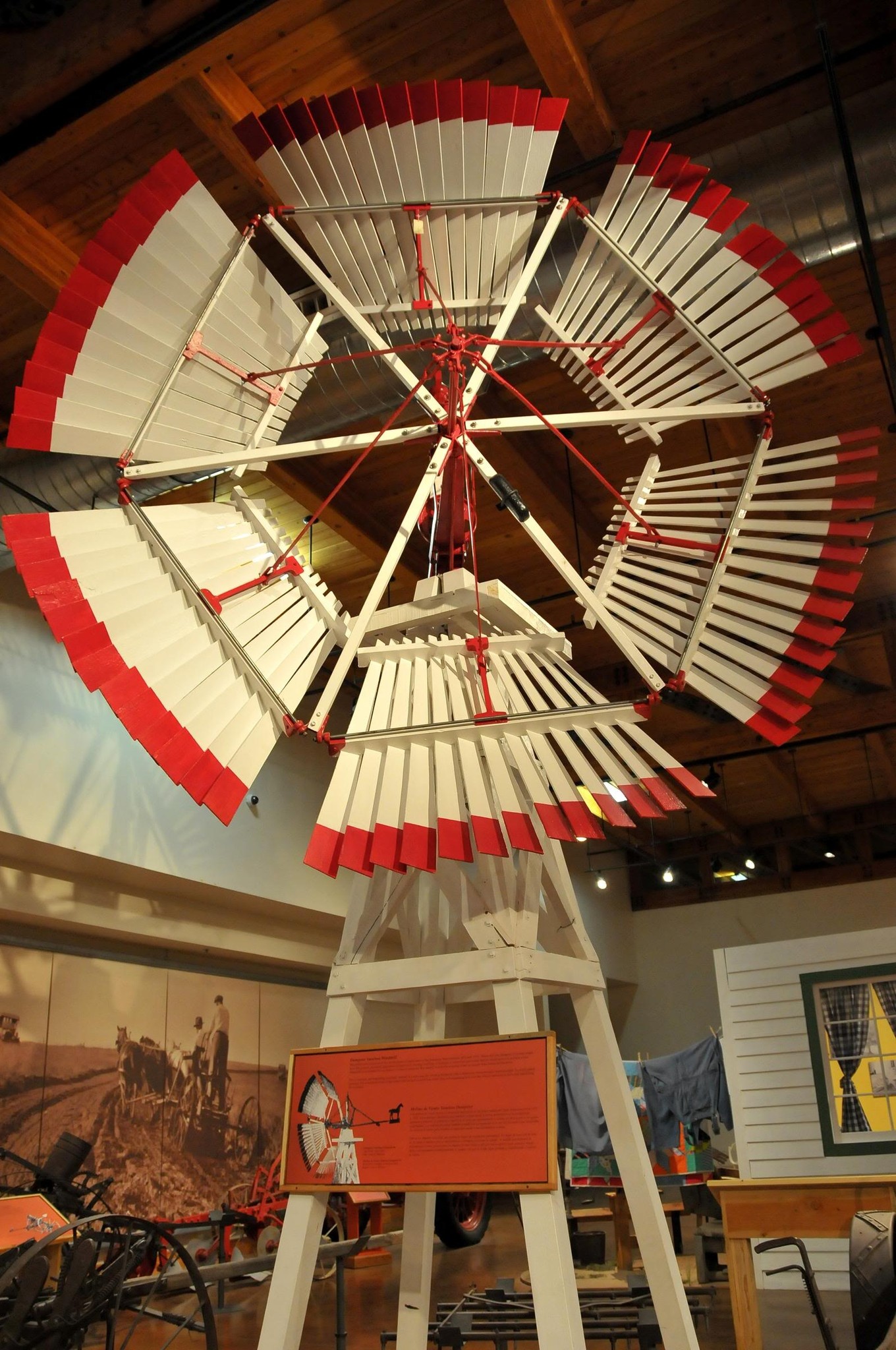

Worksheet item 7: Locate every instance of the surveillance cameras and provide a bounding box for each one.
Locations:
[246,794,259,807]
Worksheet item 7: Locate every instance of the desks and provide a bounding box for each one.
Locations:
[706,1172,896,1350]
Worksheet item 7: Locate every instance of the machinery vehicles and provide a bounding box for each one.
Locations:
[0,1132,493,1350]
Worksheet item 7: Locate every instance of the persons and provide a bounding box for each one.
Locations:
[193,1017,209,1093]
[205,993,229,1110]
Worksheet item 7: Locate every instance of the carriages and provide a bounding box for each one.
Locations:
[114,1025,262,1168]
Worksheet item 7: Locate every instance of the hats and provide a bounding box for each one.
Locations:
[214,995,223,1003]
[194,1016,204,1026]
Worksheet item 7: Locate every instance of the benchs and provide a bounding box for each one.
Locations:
[567,1203,691,1254]
[693,1219,730,1284]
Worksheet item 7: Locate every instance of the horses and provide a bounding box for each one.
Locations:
[116,1026,193,1119]
